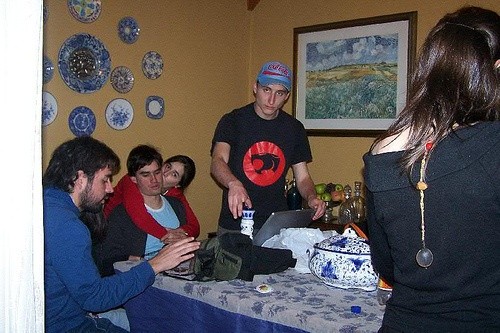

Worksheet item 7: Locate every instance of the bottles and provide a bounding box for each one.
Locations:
[287,177,302,210]
[352,181,366,218]
[339,184,356,225]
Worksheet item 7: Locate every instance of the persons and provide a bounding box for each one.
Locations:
[43,135,201,333]
[360,5,500,333]
[209,60,327,238]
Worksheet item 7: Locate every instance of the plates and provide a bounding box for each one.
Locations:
[44,56,54,85]
[43,90,58,126]
[142,51,164,79]
[111,67,134,92]
[43,3,49,24]
[69,106,96,136]
[146,95,164,119]
[105,98,134,130]
[67,0,102,23]
[58,34,111,94]
[118,16,140,44]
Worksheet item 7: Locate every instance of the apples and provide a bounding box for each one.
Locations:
[315,183,343,201]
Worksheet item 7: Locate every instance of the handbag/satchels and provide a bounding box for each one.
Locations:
[163,233,297,282]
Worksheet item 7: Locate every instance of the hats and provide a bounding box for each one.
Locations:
[257,61,294,91]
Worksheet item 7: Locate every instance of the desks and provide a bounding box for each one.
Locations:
[310,219,369,238]
[114,258,393,333]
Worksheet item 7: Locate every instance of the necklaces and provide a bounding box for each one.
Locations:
[414,137,434,268]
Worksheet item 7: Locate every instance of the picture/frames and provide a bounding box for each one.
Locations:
[292,11,418,137]
